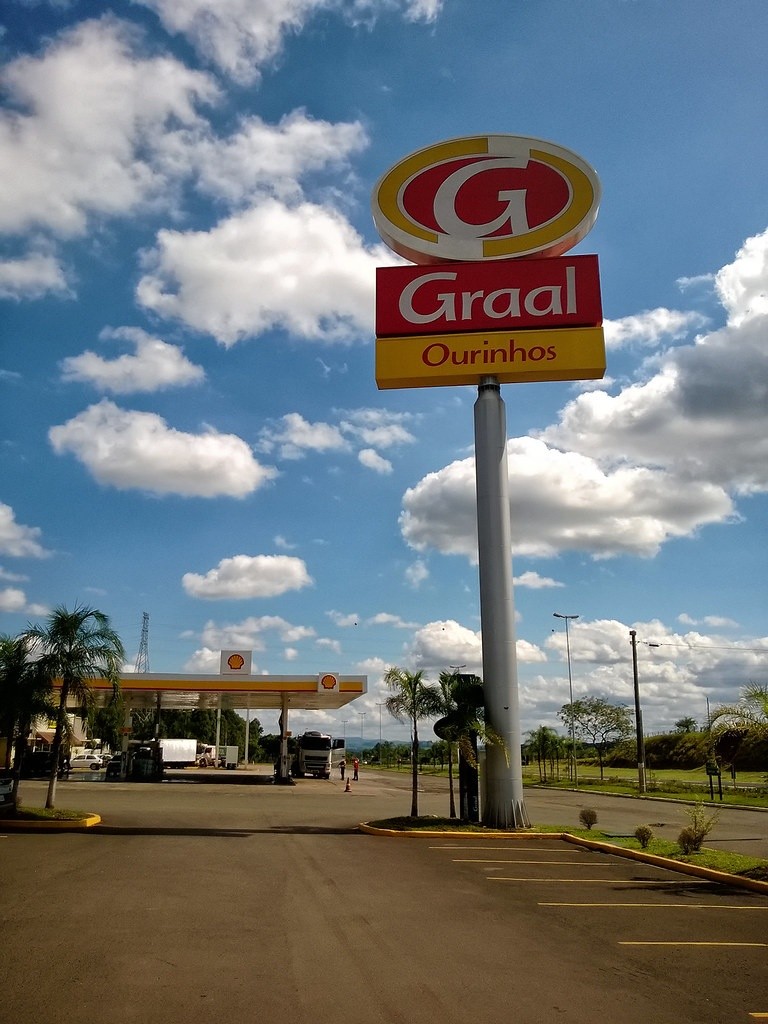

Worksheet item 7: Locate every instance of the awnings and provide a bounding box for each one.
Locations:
[37,731,80,744]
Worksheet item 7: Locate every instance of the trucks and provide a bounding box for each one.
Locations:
[139,737,198,769]
[199,745,239,770]
[190,743,208,767]
[290,731,346,779]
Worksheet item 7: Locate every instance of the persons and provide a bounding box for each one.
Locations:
[352,757,360,780]
[336,759,347,781]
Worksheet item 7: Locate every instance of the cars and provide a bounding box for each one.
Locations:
[106,755,126,777]
[63,753,104,770]
[96,754,114,767]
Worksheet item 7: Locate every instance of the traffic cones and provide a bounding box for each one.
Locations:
[344,777,352,792]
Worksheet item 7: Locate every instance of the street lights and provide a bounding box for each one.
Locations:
[375,702,388,765]
[553,613,580,786]
[341,720,349,739]
[358,712,366,762]
[450,664,467,764]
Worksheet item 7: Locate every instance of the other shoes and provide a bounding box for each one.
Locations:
[355,779,358,780]
[352,778,355,780]
[340,778,344,781]
